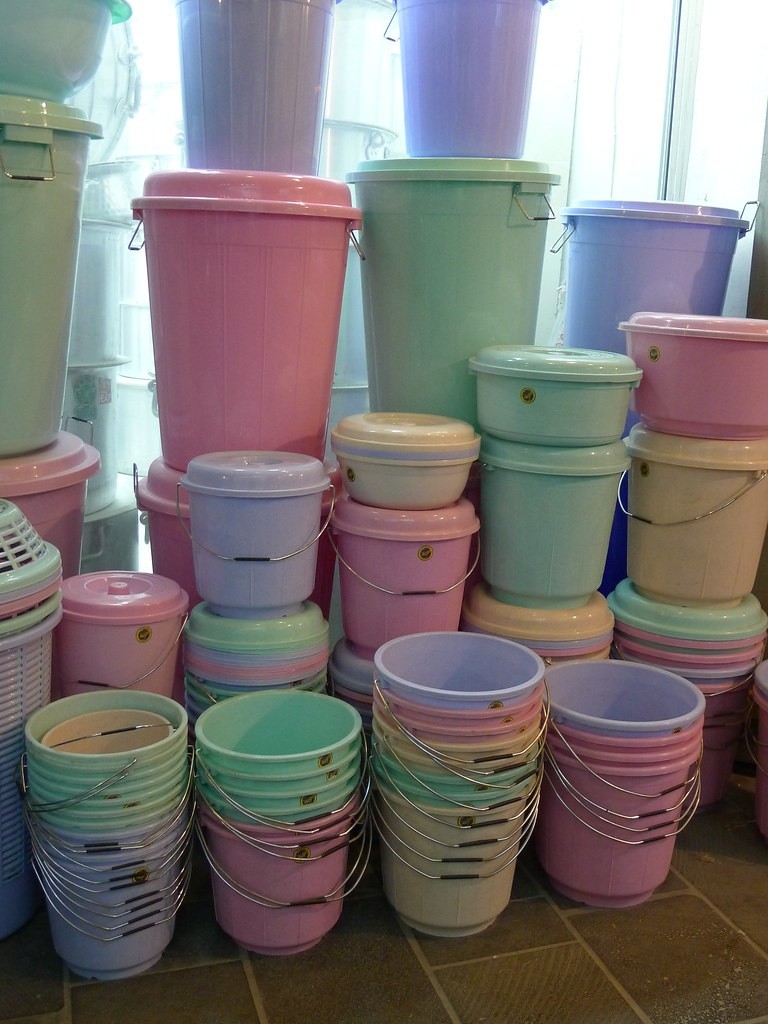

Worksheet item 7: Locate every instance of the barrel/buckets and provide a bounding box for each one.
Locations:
[184,610,374,956]
[327,635,381,739]
[329,492,481,659]
[384,0,549,159]
[126,168,364,474]
[20,689,194,980]
[605,573,768,835]
[178,450,334,618]
[464,430,631,661]
[0,431,100,579]
[346,156,562,426]
[0,95,105,460]
[175,0,343,178]
[550,199,761,351]
[133,455,344,611]
[618,423,768,610]
[537,659,706,908]
[370,631,550,939]
[52,570,190,704]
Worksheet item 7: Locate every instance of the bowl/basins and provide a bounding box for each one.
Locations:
[617,312,768,442]
[332,411,482,506]
[0,0,132,103]
[465,344,644,448]
